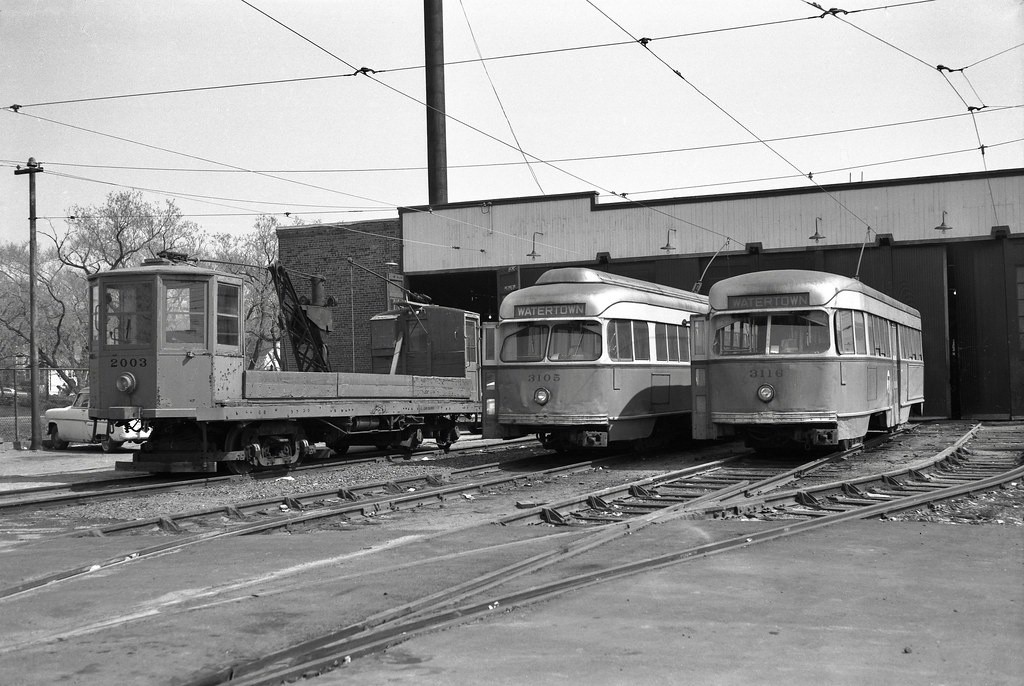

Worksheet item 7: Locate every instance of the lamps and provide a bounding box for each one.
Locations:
[525,231,544,260]
[659,228,677,253]
[934,210,953,234]
[808,216,826,243]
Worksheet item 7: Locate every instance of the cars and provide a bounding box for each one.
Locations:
[46,387,153,453]
[0,387,15,395]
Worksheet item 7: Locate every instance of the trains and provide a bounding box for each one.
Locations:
[86,258,496,476]
[690,269,924,452]
[482,268,710,450]
